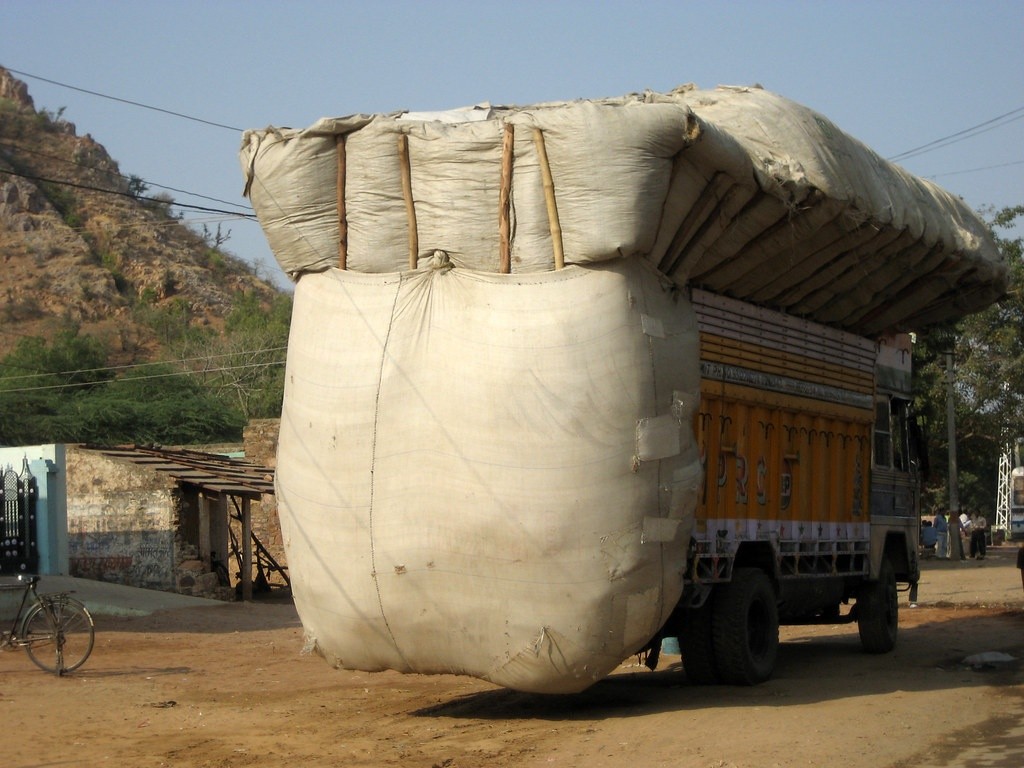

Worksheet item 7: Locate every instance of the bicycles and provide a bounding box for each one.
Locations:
[0,572,94,678]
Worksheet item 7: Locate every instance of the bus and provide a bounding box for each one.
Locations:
[1009,466,1024,533]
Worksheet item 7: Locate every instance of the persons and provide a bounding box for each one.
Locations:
[921,507,986,560]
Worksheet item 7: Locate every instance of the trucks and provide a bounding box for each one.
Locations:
[682,287,921,686]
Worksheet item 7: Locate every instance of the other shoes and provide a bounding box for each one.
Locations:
[969,553,985,559]
[935,555,950,560]
